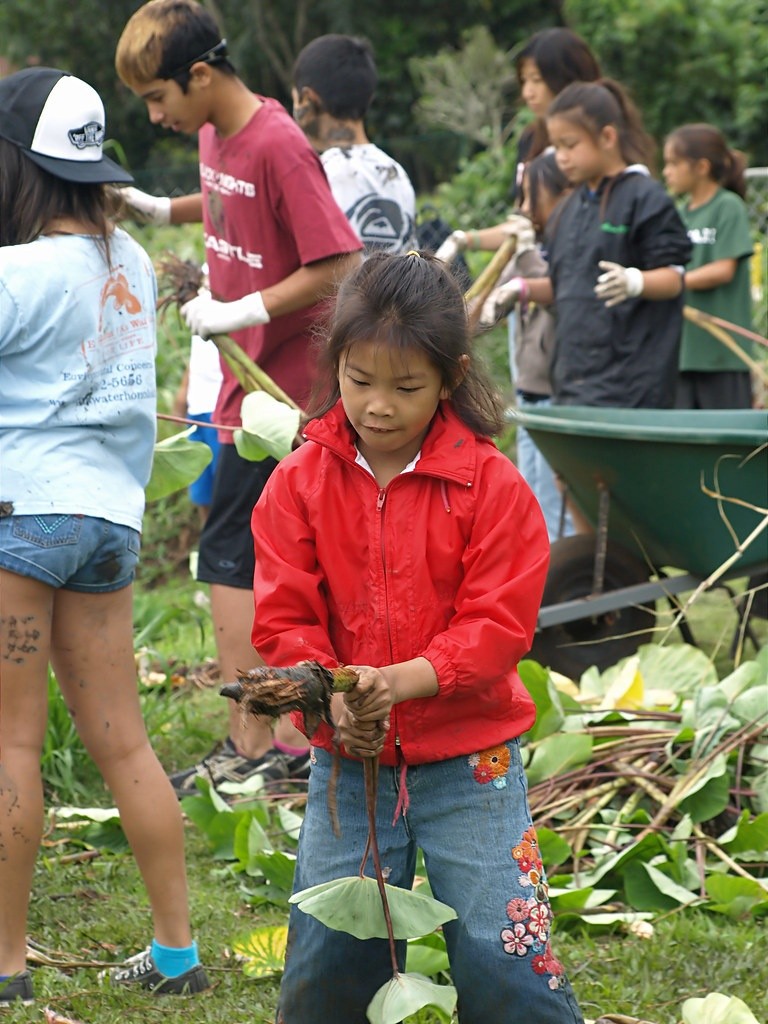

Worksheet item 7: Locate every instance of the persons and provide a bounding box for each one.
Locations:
[454,28,756,543]
[248,250,575,1024]
[0,67,213,1009]
[290,33,419,252]
[114,0,365,814]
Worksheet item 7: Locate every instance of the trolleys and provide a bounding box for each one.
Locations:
[505,397,768,681]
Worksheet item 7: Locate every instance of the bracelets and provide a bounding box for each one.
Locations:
[469,228,480,253]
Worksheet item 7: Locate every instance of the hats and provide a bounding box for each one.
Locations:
[0,66,134,187]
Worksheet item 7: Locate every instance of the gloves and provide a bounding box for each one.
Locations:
[481,275,528,326]
[118,189,171,228]
[595,258,643,309]
[178,290,270,341]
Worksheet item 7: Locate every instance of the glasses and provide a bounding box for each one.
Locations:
[176,37,231,72]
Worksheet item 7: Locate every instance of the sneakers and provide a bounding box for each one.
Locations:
[98,952,211,1002]
[0,971,34,1007]
[170,737,311,796]
